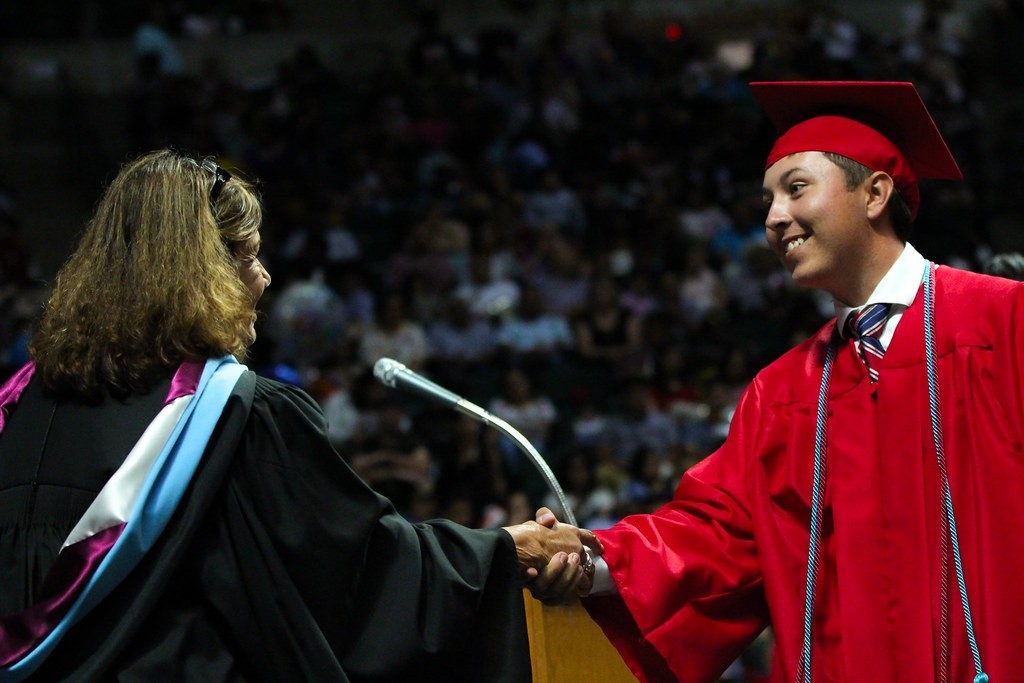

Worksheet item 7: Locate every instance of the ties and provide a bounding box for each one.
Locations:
[845,303,892,383]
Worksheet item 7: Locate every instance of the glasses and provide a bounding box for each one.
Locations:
[166,144,232,213]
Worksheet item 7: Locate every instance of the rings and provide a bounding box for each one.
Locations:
[583,553,593,570]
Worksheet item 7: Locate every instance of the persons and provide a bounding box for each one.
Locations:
[0,151,604,683]
[0,0,1024,528]
[521,77,1024,683]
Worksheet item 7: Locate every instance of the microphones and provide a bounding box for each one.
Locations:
[372,358,576,530]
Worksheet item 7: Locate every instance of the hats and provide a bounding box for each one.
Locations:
[748,81,964,222]
